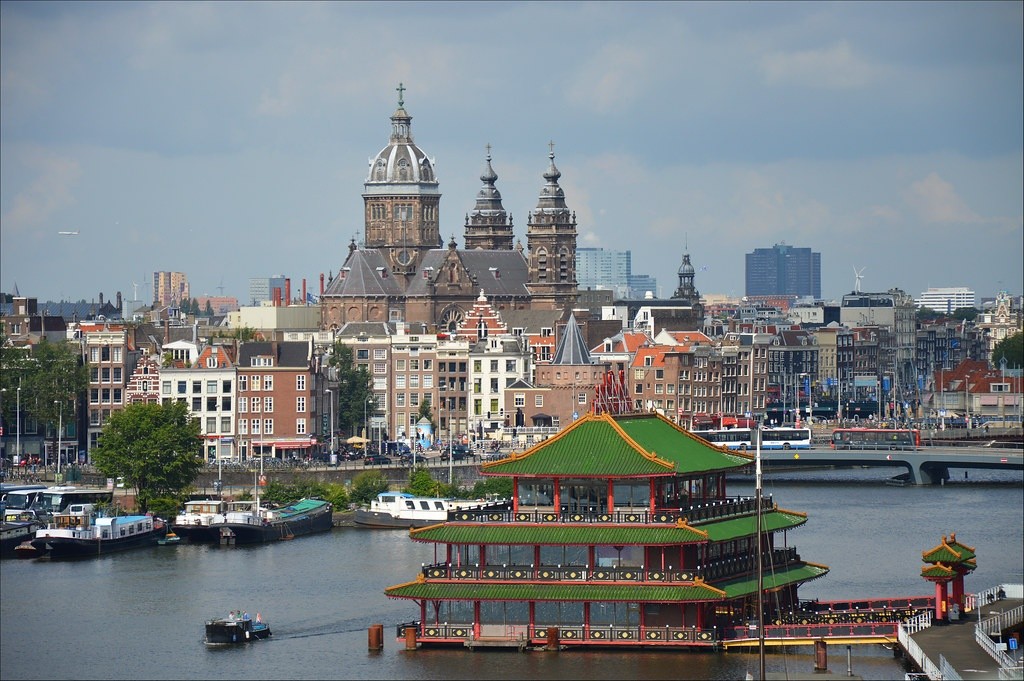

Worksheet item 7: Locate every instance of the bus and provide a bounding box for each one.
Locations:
[829,427,921,452]
[689,426,814,450]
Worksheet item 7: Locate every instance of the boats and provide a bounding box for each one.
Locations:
[202,615,274,648]
[352,488,515,530]
[0,481,181,555]
[170,456,335,545]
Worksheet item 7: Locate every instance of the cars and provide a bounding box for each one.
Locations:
[440,444,474,461]
[480,450,507,461]
[363,454,392,466]
[399,452,428,466]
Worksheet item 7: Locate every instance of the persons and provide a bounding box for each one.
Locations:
[236,610,248,619]
[229,611,235,620]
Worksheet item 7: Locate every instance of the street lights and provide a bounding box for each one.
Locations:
[965,375,971,430]
[446,399,452,485]
[16,387,22,474]
[363,394,379,458]
[54,400,63,474]
[884,370,897,429]
[799,373,813,420]
[324,389,334,455]
[941,367,951,431]
[438,384,447,453]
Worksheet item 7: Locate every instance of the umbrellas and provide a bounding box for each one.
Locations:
[341,436,371,453]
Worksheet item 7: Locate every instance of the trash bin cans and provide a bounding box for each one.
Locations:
[330,454,337,468]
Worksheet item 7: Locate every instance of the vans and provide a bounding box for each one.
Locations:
[383,441,410,455]
